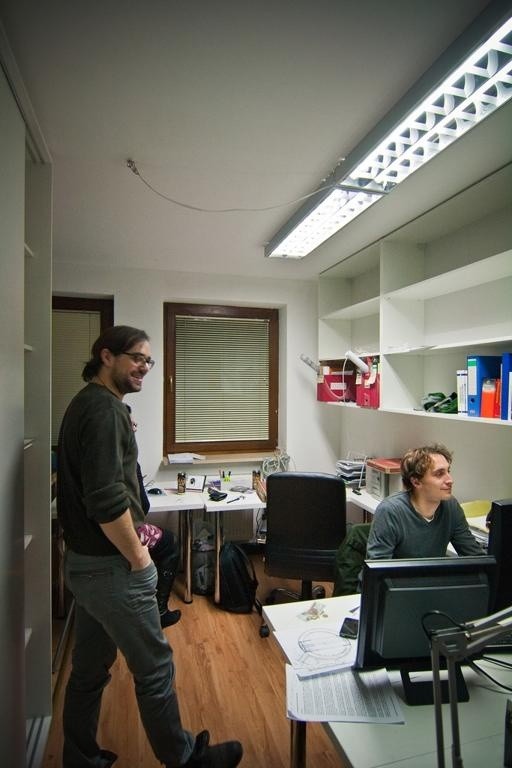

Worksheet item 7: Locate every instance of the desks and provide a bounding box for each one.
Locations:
[262,588,512,768]
[353,488,481,557]
[51,482,204,619]
[205,482,353,605]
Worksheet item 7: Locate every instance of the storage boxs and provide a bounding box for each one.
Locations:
[365,457,405,502]
[355,373,380,409]
[316,373,356,402]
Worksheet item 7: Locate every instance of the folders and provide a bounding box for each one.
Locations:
[468,355,501,417]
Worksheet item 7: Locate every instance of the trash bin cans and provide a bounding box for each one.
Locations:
[191,543,215,595]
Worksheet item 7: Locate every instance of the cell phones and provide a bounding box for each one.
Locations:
[339,618,359,639]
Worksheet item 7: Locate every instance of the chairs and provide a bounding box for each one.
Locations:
[332,523,372,594]
[244,471,356,638]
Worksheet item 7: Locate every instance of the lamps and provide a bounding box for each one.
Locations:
[263,3,512,263]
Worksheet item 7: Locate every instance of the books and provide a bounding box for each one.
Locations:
[167,453,193,463]
[456,368,468,417]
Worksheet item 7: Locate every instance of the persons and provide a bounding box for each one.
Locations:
[57,327,243,768]
[357,442,488,594]
[123,406,181,629]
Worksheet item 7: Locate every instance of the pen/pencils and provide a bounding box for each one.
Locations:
[219,471,231,481]
[227,498,240,503]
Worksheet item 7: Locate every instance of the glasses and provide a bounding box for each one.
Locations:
[122,351,154,369]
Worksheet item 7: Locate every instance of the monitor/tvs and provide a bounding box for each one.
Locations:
[352,554,496,706]
[486,498,512,650]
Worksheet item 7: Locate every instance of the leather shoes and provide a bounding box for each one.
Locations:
[161,609,180,627]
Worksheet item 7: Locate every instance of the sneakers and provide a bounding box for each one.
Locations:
[185,729,242,766]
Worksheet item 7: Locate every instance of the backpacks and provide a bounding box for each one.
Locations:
[217,539,262,615]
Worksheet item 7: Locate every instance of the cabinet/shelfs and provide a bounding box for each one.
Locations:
[315,163,512,424]
[1,25,54,768]
